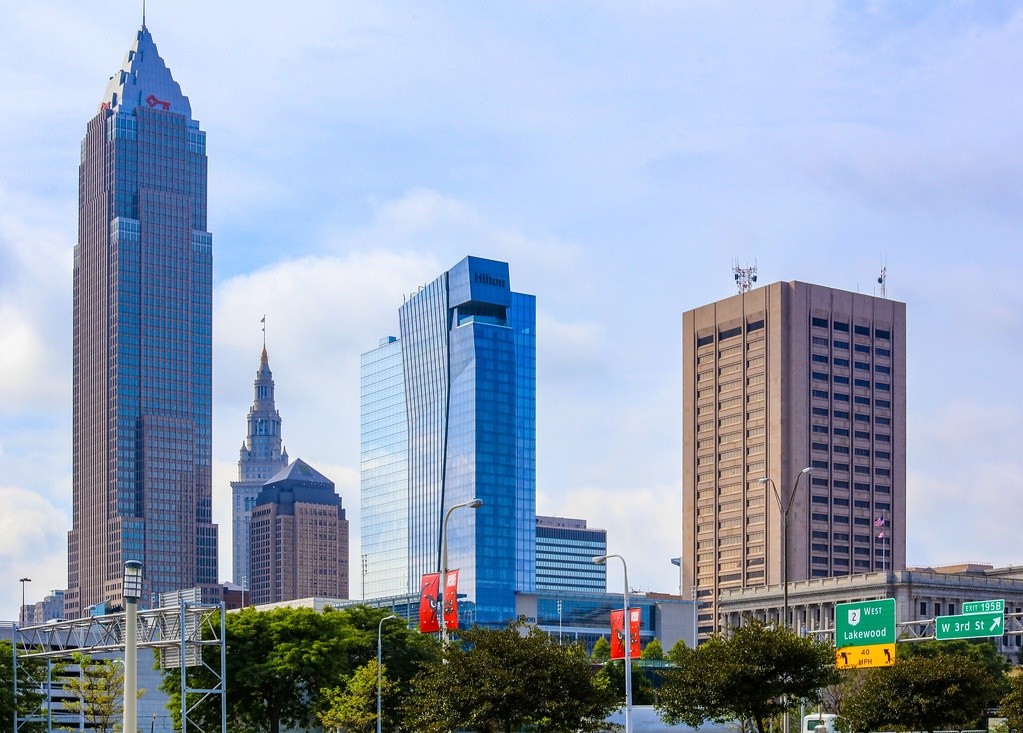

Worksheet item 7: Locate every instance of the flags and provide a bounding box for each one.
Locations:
[609,610,625,660]
[629,607,641,659]
[443,568,459,631]
[419,572,440,633]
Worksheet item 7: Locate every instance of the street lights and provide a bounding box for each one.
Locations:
[79,604,96,733]
[591,554,634,733]
[376,614,397,733]
[757,466,814,629]
[18,577,33,626]
[441,499,483,665]
[119,560,144,733]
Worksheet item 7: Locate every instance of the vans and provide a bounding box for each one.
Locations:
[802,714,851,733]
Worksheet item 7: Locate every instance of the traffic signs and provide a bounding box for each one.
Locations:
[935,612,1005,641]
[833,598,897,671]
